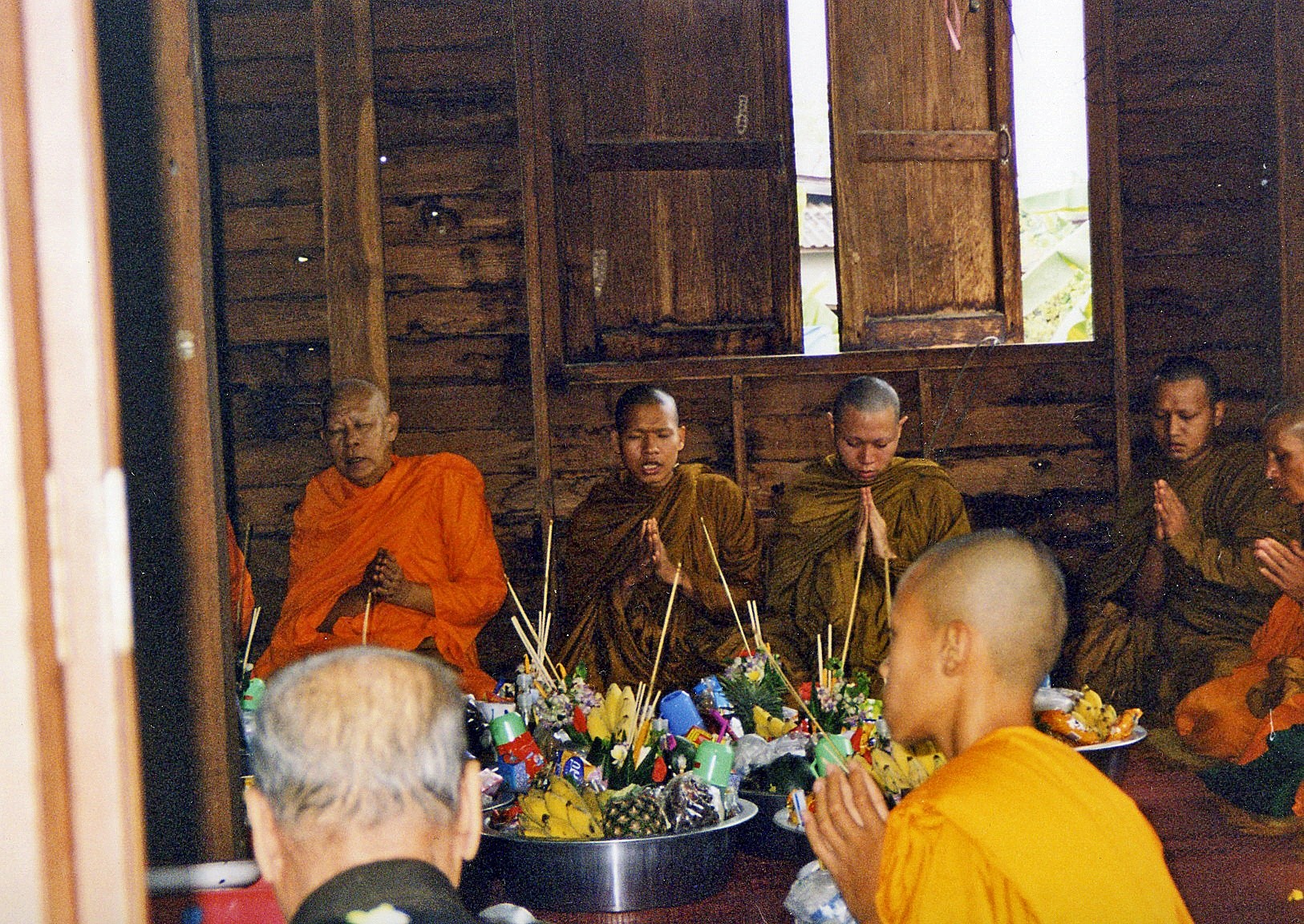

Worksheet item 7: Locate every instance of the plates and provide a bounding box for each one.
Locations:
[1073,725,1147,750]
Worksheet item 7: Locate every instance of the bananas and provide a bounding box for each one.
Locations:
[845,738,948,793]
[519,775,614,840]
[585,684,642,746]
[750,705,797,737]
[1071,683,1116,737]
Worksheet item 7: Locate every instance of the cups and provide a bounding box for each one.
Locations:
[242,679,265,709]
[492,712,527,746]
[694,675,728,708]
[658,689,703,735]
[693,740,734,788]
[810,734,853,779]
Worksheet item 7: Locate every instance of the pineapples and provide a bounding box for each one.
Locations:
[601,791,674,840]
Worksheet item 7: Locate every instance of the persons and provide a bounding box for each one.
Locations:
[546,386,759,701]
[1063,355,1304,727]
[761,373,973,699]
[249,379,515,704]
[240,646,546,924]
[1174,392,1304,816]
[799,525,1196,924]
[226,513,255,652]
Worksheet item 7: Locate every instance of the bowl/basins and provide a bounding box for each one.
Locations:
[1077,736,1146,787]
[480,790,819,911]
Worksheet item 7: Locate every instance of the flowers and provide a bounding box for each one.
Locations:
[534,664,600,742]
[720,647,792,730]
[611,724,677,790]
[813,657,870,734]
